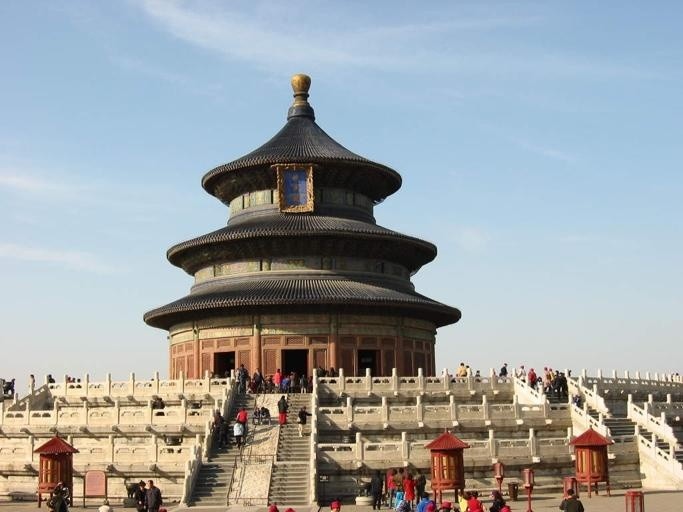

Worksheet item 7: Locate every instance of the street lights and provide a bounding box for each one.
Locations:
[492,461,505,496]
[562,476,580,506]
[521,467,536,512]
[624,489,645,512]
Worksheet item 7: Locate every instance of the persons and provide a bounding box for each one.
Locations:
[134,478,145,511]
[284,507,296,512]
[45,480,71,512]
[558,488,584,512]
[330,498,340,512]
[47,373,56,388]
[212,395,312,449]
[447,362,583,409]
[153,398,165,416]
[208,364,338,395]
[65,377,81,388]
[368,467,514,512]
[268,501,279,512]
[145,479,163,512]
[26,374,35,392]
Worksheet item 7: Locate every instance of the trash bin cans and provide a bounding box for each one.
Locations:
[508,481,519,501]
